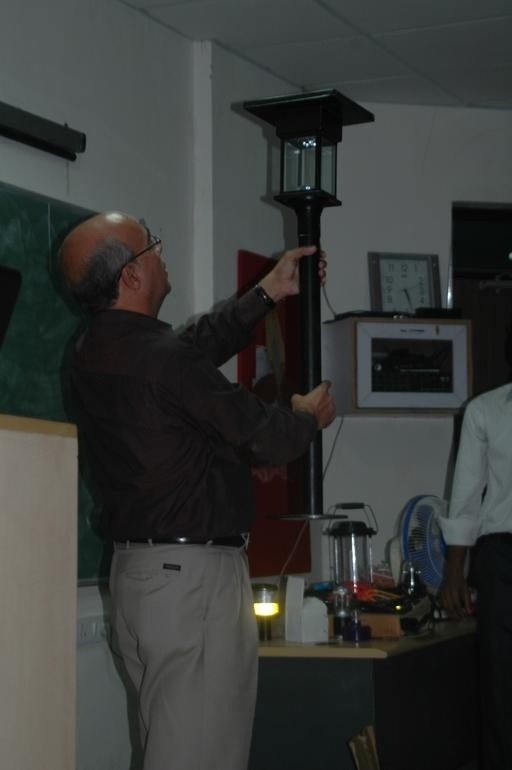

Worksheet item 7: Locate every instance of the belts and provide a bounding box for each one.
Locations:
[121,532,245,549]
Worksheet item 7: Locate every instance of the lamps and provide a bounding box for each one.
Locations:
[243,89,376,521]
[251,582,282,616]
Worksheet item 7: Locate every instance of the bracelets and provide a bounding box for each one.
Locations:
[254,284,280,312]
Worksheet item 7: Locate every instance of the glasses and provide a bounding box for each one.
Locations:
[117,234,163,285]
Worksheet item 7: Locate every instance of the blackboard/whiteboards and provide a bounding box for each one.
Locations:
[0,180,117,587]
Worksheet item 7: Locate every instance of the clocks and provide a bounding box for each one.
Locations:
[368,249,443,317]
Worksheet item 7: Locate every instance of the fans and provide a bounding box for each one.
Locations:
[386,494,450,621]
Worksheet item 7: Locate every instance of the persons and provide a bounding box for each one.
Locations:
[54,210,341,768]
[438,283,510,767]
